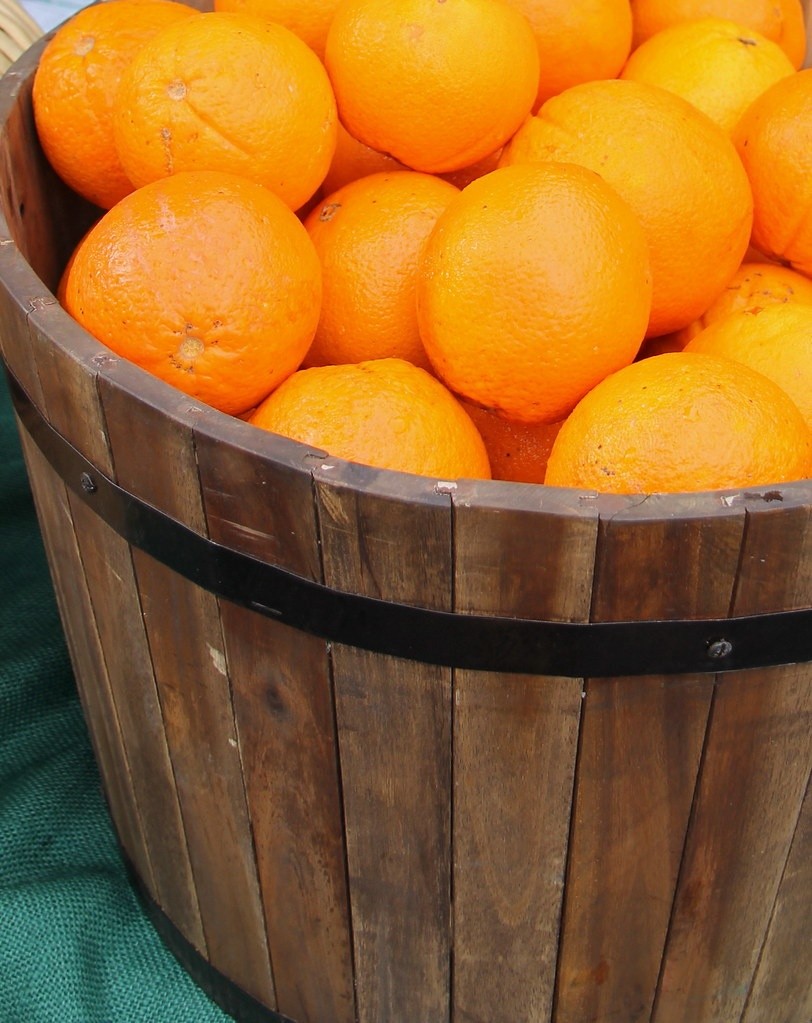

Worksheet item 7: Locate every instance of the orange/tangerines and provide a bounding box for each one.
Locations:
[31,0,812,494]
